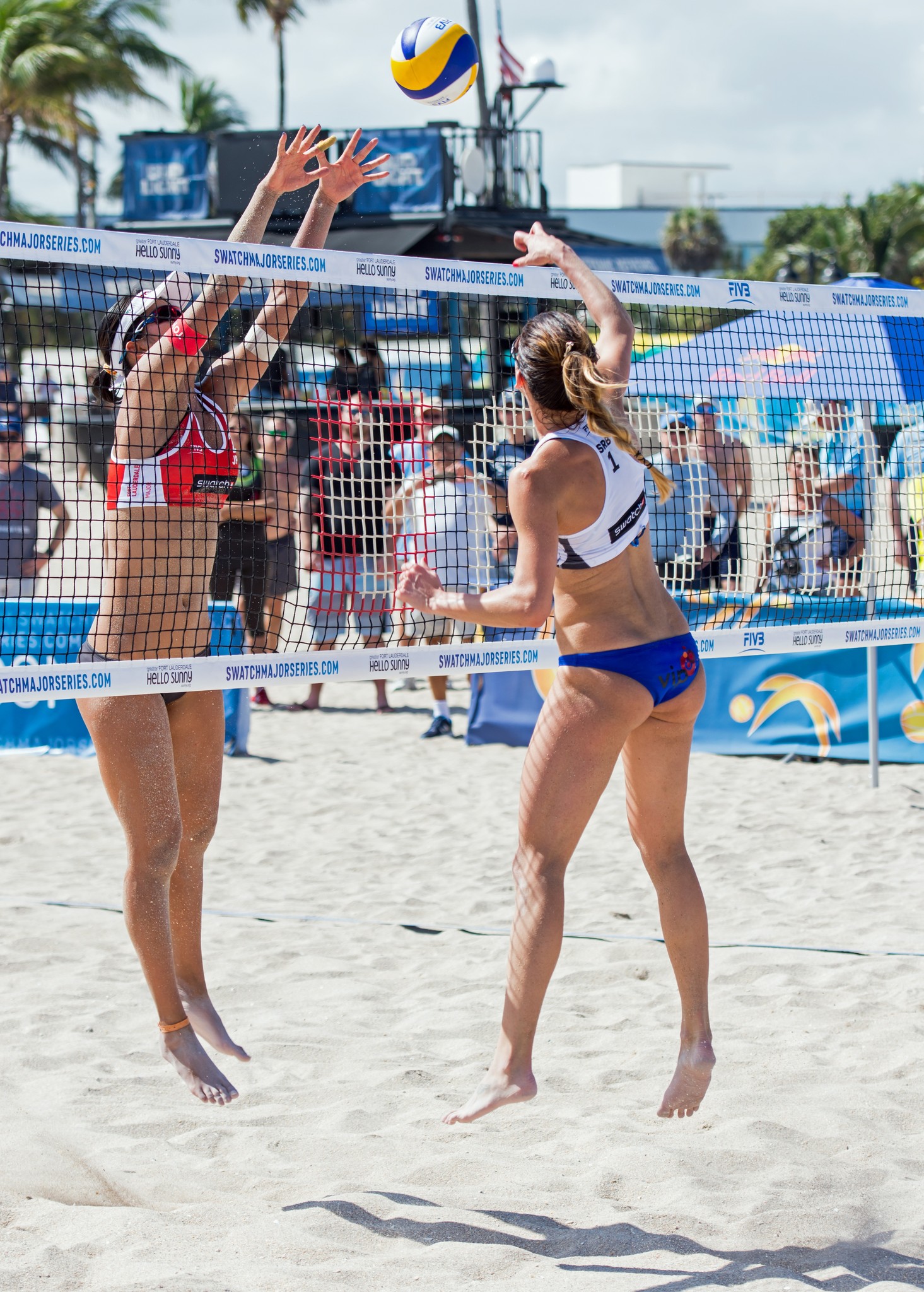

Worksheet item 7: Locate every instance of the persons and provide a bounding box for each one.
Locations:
[397,219,718,1126]
[0,334,922,736]
[70,123,392,1105]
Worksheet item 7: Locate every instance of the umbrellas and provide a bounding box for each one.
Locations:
[625,271,924,788]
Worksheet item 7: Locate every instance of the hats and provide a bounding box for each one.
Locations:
[801,396,831,425]
[427,425,459,443]
[414,396,446,417]
[0,415,24,435]
[499,387,528,410]
[692,395,716,411]
[109,269,194,374]
[660,409,693,429]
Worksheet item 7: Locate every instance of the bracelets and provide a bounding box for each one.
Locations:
[46,548,54,557]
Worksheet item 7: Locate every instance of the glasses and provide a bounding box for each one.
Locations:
[697,405,718,414]
[347,409,369,417]
[229,426,250,433]
[266,430,290,440]
[118,305,183,364]
[665,425,687,436]
[510,334,526,376]
[501,404,521,413]
[0,435,19,443]
[423,413,441,419]
[792,443,815,452]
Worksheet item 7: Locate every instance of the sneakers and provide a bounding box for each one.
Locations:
[421,716,452,738]
[250,689,274,709]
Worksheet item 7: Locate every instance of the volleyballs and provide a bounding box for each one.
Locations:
[391,16,478,107]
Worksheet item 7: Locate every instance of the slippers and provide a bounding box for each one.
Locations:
[377,704,393,713]
[289,701,312,710]
